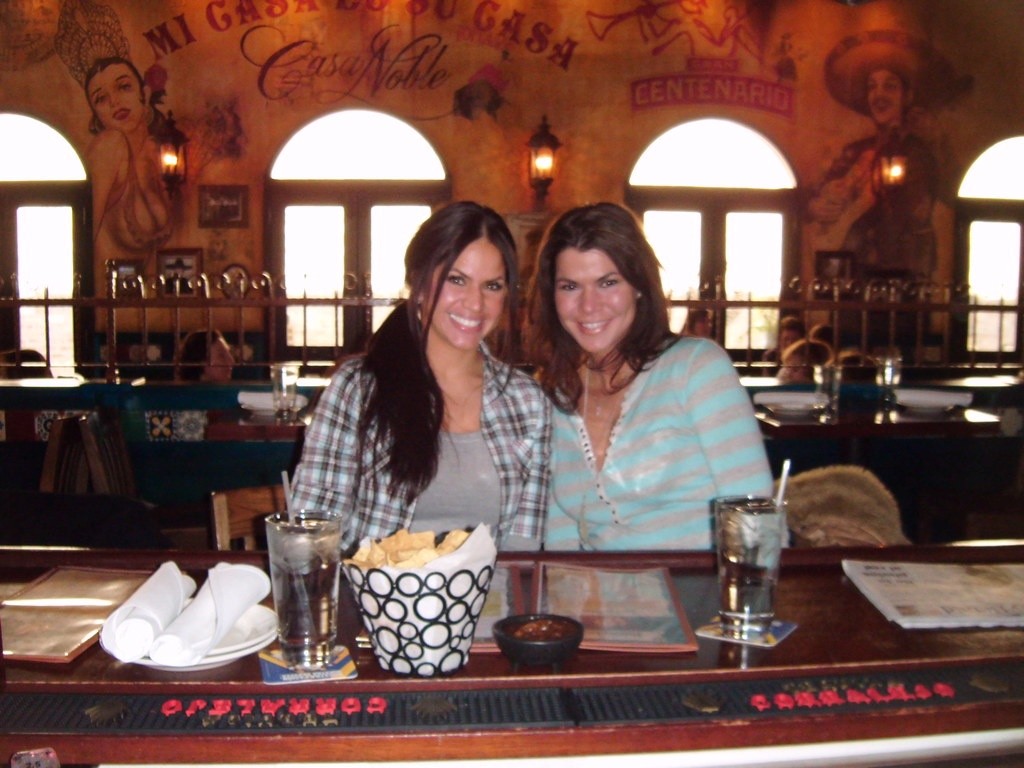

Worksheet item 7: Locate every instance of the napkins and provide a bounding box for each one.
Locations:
[894,388,974,407]
[753,390,829,407]
[100,559,200,664]
[149,560,271,668]
[237,391,308,413]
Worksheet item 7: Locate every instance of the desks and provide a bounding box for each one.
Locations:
[204,405,310,443]
[755,407,1000,467]
[0,545,1023,768]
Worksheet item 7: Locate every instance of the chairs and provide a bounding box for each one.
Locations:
[0,350,1024,552]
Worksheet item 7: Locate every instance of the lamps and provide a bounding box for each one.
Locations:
[872,145,907,205]
[157,110,192,197]
[527,112,562,200]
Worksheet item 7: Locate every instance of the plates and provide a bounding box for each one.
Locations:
[891,392,973,414]
[239,392,309,417]
[99,599,278,672]
[763,396,826,418]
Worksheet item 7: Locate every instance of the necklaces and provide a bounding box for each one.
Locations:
[583,364,621,456]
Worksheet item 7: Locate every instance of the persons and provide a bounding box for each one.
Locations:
[763,318,876,384]
[178,330,234,380]
[525,203,790,552]
[290,201,552,553]
[681,309,712,336]
[0,350,53,379]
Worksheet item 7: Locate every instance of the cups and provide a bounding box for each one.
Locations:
[264,511,344,665]
[875,357,901,411]
[815,363,843,411]
[270,364,302,422]
[712,494,790,639]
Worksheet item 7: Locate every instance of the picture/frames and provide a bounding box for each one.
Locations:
[814,249,853,298]
[112,260,144,299]
[198,184,247,228]
[155,246,202,297]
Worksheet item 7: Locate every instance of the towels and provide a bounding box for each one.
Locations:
[99,560,197,664]
[149,561,271,664]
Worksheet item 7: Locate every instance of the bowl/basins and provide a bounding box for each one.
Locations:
[493,613,584,667]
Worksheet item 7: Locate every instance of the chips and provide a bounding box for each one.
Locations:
[340,528,474,565]
